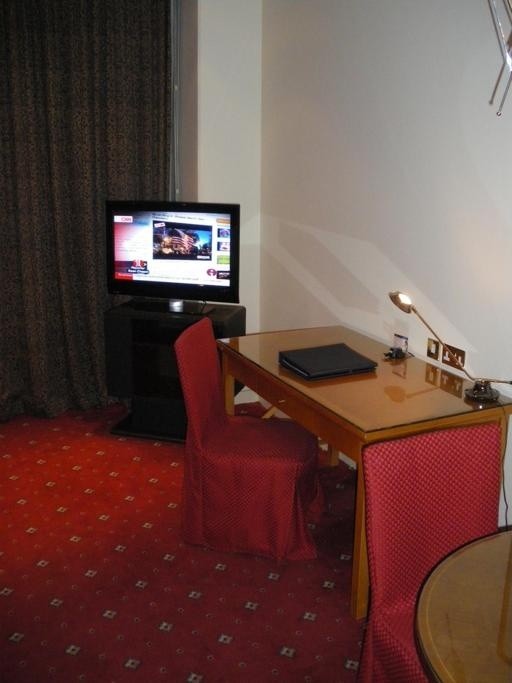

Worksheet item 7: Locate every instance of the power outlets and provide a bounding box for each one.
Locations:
[443,346,466,372]
[441,371,463,399]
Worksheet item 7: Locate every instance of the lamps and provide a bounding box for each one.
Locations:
[389,291,499,404]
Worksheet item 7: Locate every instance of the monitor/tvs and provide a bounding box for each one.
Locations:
[105,199,240,315]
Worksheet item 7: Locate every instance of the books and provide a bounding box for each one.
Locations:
[278,341,379,382]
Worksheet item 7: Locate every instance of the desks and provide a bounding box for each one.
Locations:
[414,525,512,683]
[215,327,512,620]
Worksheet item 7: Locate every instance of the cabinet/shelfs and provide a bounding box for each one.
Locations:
[104,298,245,443]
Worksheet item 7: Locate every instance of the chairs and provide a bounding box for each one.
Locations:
[175,318,324,565]
[360,421,501,683]
